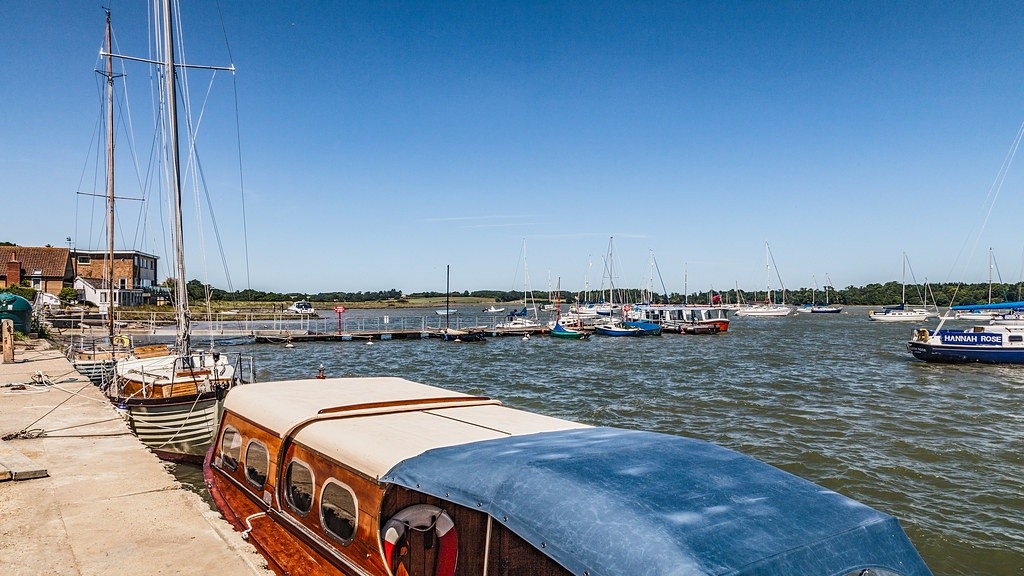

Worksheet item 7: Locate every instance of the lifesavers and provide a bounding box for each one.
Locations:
[918,328,930,342]
[380,505,458,576]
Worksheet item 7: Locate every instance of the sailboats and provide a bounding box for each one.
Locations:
[90,0,260,465]
[495,237,542,329]
[64,6,171,384]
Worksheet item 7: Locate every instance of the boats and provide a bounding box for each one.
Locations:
[484,308,505,312]
[436,309,458,315]
[202,373,932,576]
[440,328,487,342]
[542,231,1024,367]
[286,300,318,317]
